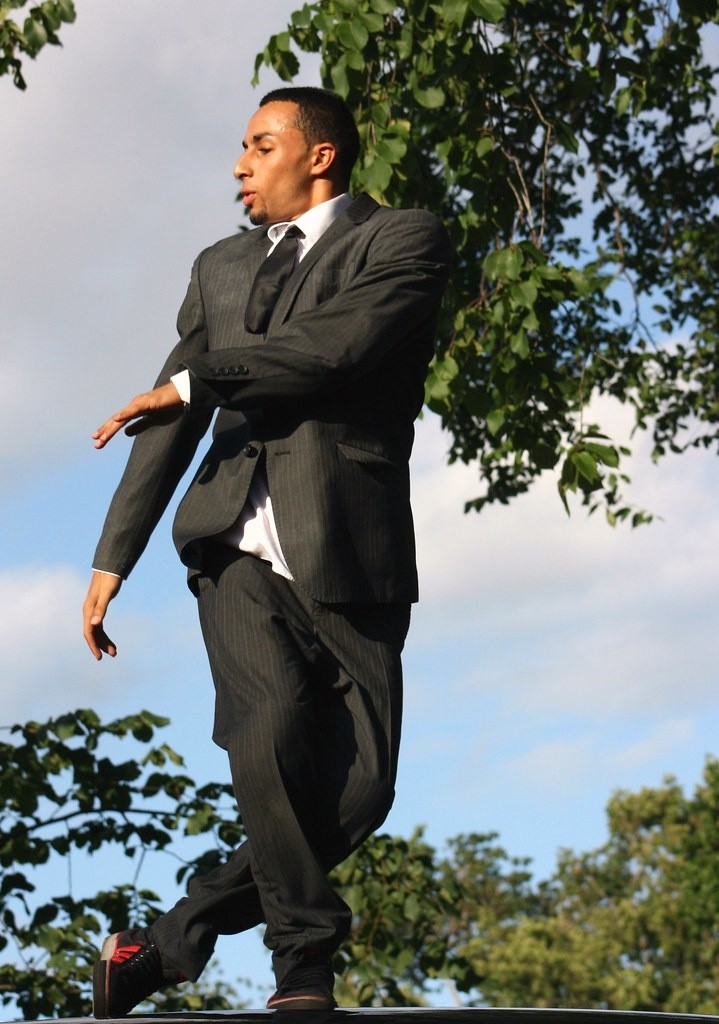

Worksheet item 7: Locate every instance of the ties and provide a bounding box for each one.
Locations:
[244,227,306,334]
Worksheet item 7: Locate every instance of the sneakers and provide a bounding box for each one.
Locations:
[93,929,168,1020]
[266,962,338,1010]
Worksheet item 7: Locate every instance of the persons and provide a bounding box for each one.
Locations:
[83,87,450,1020]
